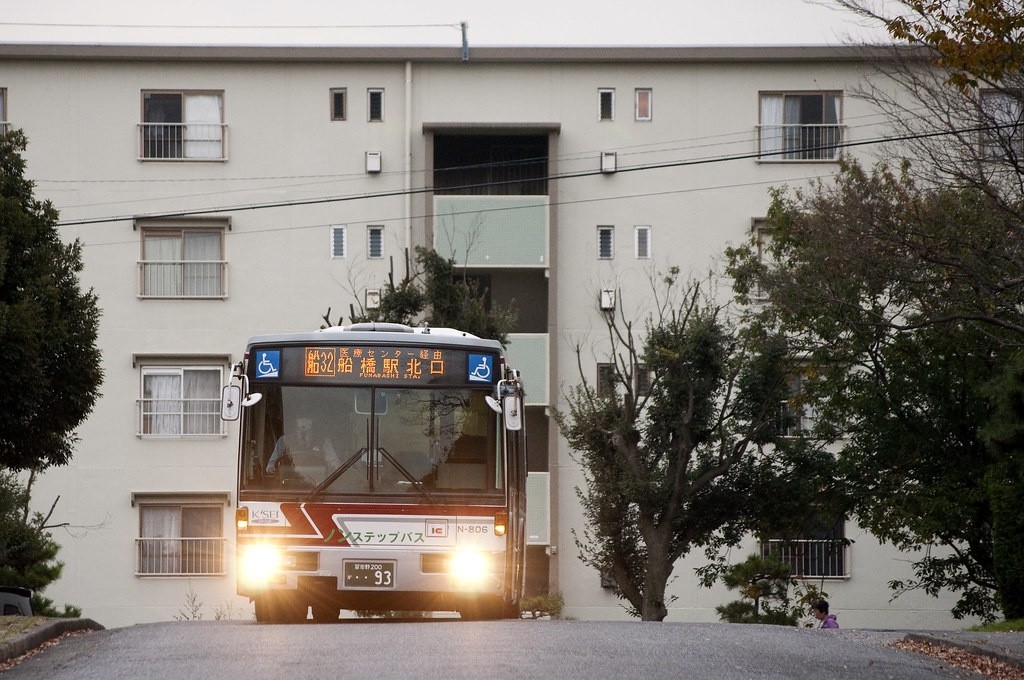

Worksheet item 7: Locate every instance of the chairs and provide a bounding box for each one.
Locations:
[452,432,487,460]
[392,452,432,481]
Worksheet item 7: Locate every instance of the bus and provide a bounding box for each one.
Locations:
[220,323,528,620]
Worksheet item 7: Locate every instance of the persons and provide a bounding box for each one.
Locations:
[812,600,839,628]
[266,411,341,477]
